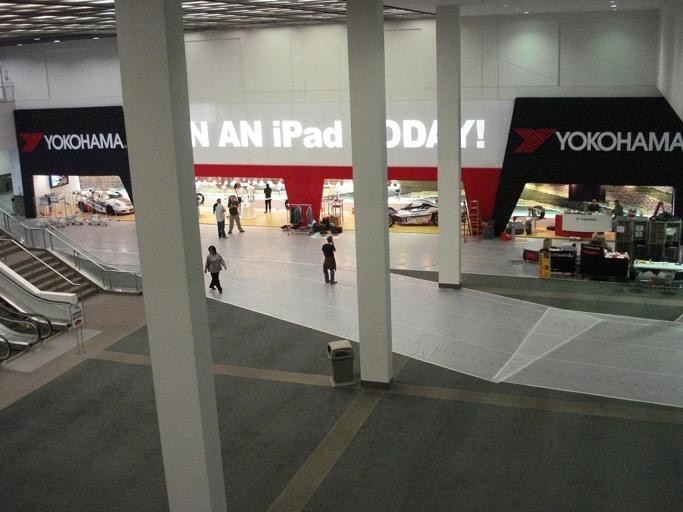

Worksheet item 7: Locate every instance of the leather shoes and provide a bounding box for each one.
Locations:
[228,232,232,234]
[326,280,337,284]
[240,230,245,232]
[209,286,222,294]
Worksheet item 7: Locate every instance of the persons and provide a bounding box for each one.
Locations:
[225,195,245,234]
[320,236,338,285]
[203,246,227,294]
[212,198,229,239]
[609,199,623,231]
[232,181,257,202]
[264,183,272,213]
[588,198,601,210]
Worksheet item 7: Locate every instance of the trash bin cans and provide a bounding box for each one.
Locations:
[326,340,355,385]
[538,247,551,279]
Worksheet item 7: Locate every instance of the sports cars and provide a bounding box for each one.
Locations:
[387,202,439,227]
[76,192,134,215]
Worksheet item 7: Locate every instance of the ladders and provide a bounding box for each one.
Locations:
[464,200,479,243]
